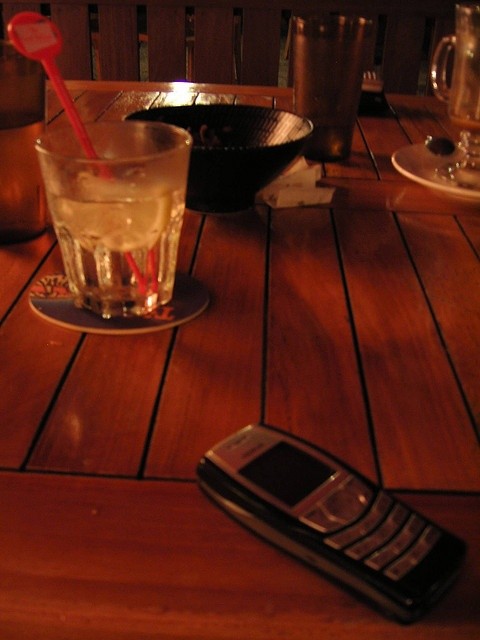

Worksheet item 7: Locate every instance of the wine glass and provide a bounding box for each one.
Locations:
[429,5,480,192]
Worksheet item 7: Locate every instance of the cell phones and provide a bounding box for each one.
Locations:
[196,422,468,625]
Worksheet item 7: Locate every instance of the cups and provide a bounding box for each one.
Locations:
[293,14,371,162]
[33,123,192,316]
[1,38,49,244]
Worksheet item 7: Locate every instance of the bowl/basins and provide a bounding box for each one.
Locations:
[123,104,314,213]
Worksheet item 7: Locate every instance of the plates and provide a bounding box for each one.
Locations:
[392,142,480,204]
[27,273,213,336]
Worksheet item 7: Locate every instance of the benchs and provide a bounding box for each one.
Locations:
[0,3,458,95]
